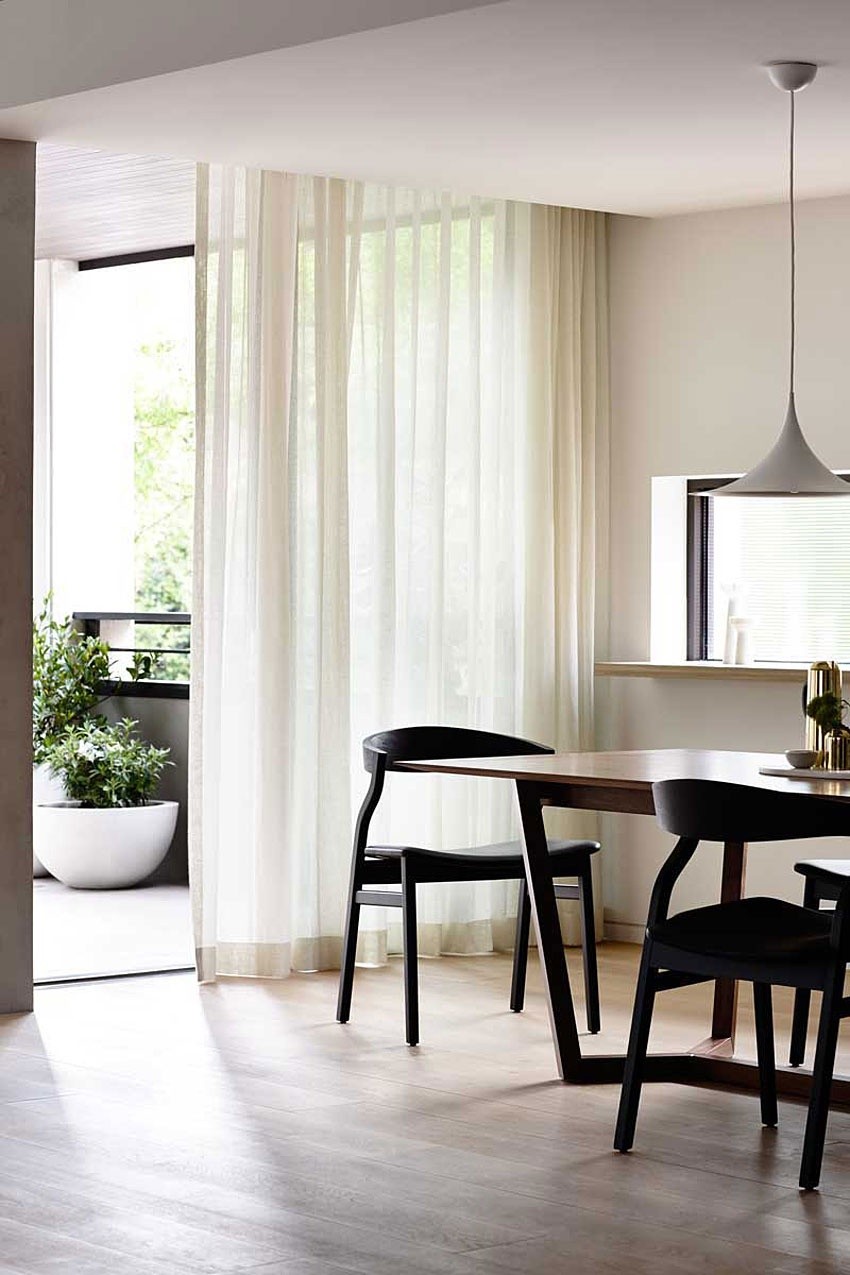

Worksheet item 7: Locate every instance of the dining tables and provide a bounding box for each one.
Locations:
[390,749,850,1110]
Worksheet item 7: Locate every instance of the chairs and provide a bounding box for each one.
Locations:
[613,779,850,1193]
[789,858,850,1071]
[334,725,600,1045]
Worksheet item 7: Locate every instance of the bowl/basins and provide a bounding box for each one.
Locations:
[784,750,818,769]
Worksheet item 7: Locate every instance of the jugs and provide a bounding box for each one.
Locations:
[800,660,842,768]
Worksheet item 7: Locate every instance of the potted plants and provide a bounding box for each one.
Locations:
[32,588,179,891]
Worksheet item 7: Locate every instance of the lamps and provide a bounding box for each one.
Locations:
[688,61,849,497]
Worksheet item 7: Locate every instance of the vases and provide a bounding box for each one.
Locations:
[31,761,77,878]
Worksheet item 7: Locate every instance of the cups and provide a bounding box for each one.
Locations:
[822,728,849,770]
